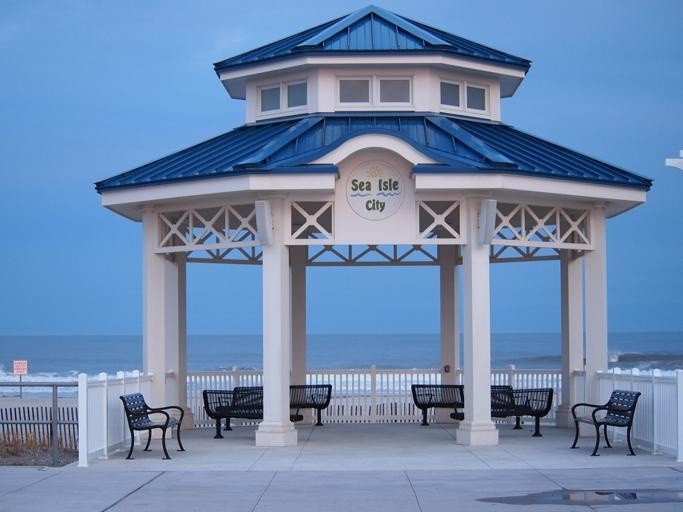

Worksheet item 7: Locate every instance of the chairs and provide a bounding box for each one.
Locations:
[570,390,642,456]
[119,392,186,460]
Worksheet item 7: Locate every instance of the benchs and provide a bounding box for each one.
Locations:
[411,384,554,437]
[202,384,332,439]
